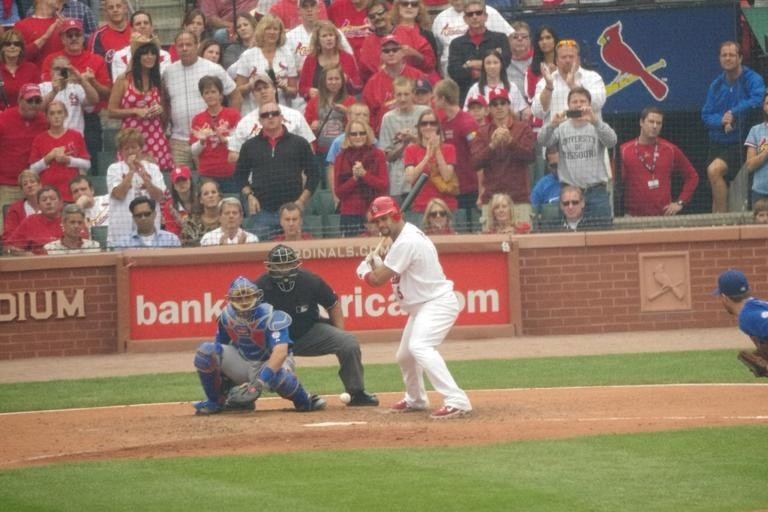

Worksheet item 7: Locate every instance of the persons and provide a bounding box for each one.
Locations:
[713,268,768,377]
[192,275,327,415]
[357,197,472,419]
[701,40,768,224]
[253,244,379,406]
[1,0,617,254]
[616,105,701,216]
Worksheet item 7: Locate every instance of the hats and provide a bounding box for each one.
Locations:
[59,19,83,33]
[489,89,511,103]
[20,84,42,100]
[130,33,160,55]
[171,166,191,182]
[713,269,749,295]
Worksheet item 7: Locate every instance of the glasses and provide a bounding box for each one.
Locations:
[2,40,20,47]
[258,1,484,137]
[133,211,152,218]
[562,200,580,206]
[430,210,447,218]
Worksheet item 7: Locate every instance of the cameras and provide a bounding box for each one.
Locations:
[567,110,581,119]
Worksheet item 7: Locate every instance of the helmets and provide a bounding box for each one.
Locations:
[265,244,302,296]
[368,197,401,222]
[225,277,264,324]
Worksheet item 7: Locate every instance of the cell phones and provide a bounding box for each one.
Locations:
[61,68,67,77]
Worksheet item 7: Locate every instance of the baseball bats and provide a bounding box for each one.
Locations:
[359,173,429,281]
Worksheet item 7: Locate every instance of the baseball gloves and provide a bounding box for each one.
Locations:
[227,381,262,406]
[738,351,768,377]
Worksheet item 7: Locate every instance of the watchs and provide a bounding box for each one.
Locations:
[676,200,686,208]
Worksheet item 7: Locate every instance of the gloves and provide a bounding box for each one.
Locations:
[356,261,372,281]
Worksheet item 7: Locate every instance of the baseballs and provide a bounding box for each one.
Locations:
[341,392,351,403]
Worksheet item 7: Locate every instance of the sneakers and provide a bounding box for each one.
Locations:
[296,395,325,411]
[347,391,380,407]
[195,398,225,414]
[430,405,461,419]
[390,400,412,412]
